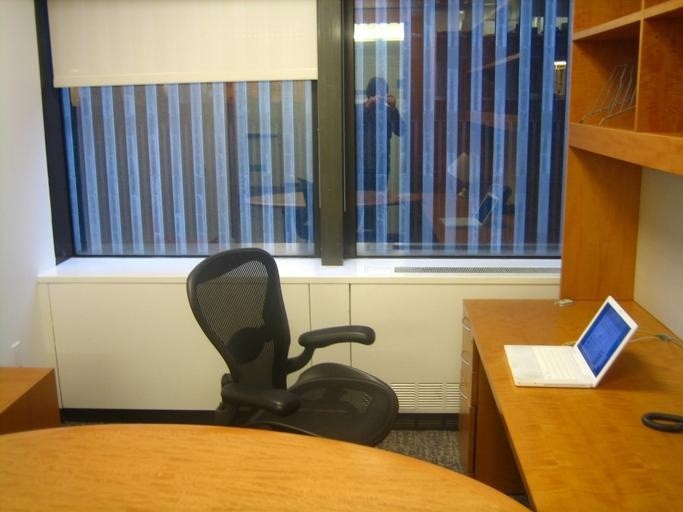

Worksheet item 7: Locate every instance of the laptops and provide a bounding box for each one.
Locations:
[502,295,638,389]
[440,193,499,228]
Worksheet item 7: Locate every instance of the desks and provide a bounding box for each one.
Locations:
[463,298,682,512]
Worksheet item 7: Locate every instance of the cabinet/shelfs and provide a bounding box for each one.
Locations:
[566,1,682,172]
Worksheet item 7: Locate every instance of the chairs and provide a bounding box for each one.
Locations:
[186,248,398,451]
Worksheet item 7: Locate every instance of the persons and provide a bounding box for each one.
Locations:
[353,77,403,192]
